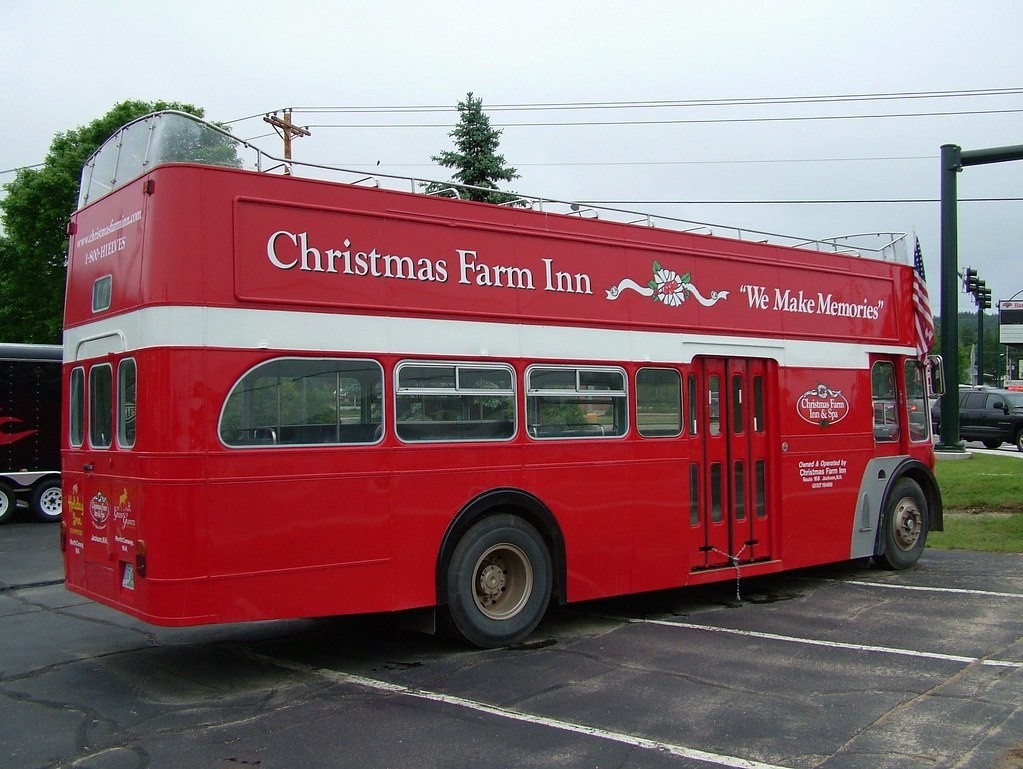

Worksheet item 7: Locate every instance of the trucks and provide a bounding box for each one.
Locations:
[0,342,114,523]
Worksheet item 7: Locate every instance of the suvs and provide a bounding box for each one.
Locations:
[929,385,1023,452]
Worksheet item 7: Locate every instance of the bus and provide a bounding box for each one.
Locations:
[60,109,946,650]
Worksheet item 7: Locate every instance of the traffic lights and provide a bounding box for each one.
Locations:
[966,268,976,293]
[977,277,985,306]
[985,288,991,309]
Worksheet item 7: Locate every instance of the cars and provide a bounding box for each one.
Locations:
[873,401,922,441]
[887,398,936,422]
